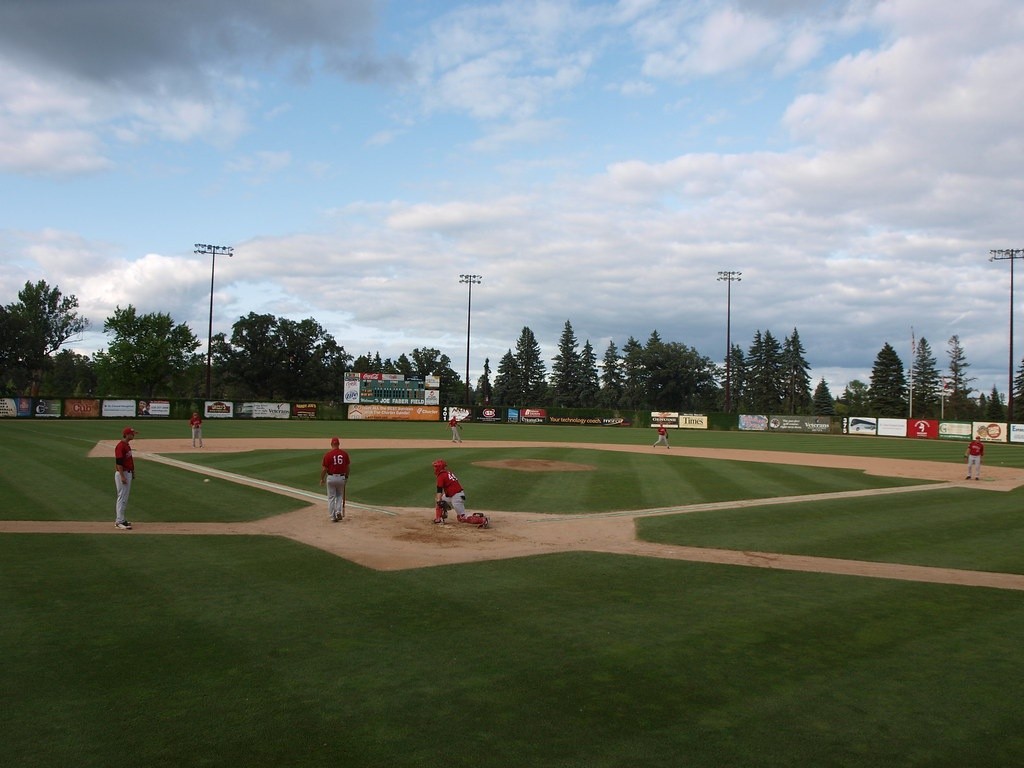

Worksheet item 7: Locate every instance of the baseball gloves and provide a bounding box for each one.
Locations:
[437,500,448,512]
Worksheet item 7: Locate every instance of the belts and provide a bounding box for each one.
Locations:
[458,490,464,493]
[117,470,130,473]
[329,473,345,476]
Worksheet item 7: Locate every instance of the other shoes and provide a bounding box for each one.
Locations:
[434,519,444,525]
[478,518,490,529]
[976,478,978,480]
[337,513,342,519]
[966,476,971,480]
[333,519,338,522]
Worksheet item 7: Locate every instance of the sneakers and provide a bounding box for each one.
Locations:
[116,524,132,529]
[115,522,131,527]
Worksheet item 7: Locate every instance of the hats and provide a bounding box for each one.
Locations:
[193,413,198,418]
[332,438,339,443]
[976,436,980,439]
[123,428,138,434]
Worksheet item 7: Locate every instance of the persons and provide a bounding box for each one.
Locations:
[964,435,984,481]
[432,460,491,528]
[115,427,139,529]
[320,437,350,523]
[446,416,464,443]
[653,421,671,449]
[190,413,204,449]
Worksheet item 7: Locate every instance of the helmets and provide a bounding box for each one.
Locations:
[432,459,447,476]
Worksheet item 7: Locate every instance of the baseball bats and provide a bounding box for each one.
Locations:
[343,484,345,517]
